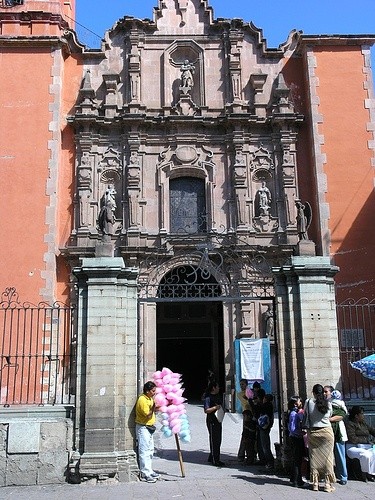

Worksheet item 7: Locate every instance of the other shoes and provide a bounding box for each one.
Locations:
[140,473,161,484]
[324,484,336,492]
[213,460,224,466]
[341,479,347,485]
[312,484,320,491]
[238,456,274,469]
[297,482,309,488]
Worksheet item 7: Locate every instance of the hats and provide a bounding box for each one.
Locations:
[331,390,341,399]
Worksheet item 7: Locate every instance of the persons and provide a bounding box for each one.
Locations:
[345,406,375,482]
[284,384,348,488]
[238,379,274,471]
[135,381,162,483]
[201,380,229,466]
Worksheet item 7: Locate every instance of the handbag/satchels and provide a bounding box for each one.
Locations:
[258,415,270,430]
[145,425,157,434]
[299,398,310,429]
[214,404,224,422]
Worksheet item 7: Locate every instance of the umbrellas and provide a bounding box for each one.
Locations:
[350,354,375,381]
